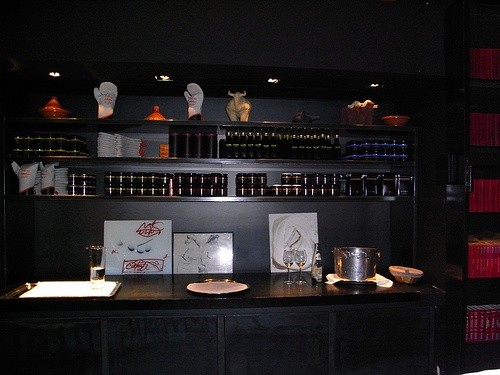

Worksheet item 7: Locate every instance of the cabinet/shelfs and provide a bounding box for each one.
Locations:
[444,0,500,375]
[0,118,444,375]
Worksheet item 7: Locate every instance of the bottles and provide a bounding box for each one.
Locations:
[311,243,323,286]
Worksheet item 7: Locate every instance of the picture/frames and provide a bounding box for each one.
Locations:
[172,231,234,275]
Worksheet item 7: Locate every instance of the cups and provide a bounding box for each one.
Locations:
[87,245,107,281]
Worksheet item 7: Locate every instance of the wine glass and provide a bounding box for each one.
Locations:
[283,250,294,285]
[294,250,307,285]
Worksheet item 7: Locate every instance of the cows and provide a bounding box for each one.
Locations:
[225,90,252,121]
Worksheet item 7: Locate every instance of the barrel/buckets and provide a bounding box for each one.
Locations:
[330,247,382,281]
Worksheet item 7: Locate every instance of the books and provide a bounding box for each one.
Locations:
[469,179,500,212]
[468,47,500,81]
[467,234,500,278]
[469,112,500,147]
[465,304,500,344]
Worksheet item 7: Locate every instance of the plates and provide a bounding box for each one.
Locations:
[184,278,251,297]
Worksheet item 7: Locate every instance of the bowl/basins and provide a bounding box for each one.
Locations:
[382,116,409,126]
